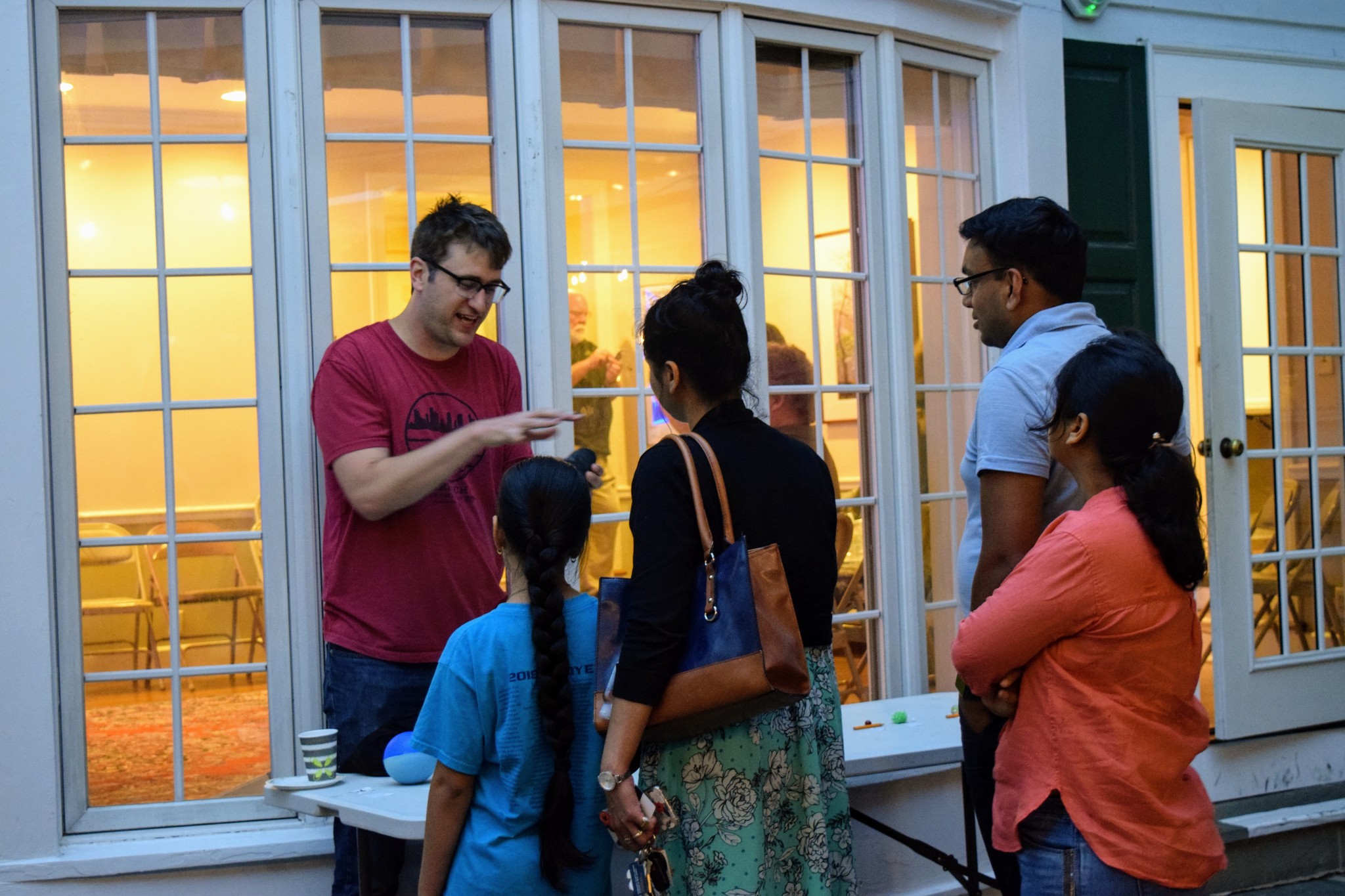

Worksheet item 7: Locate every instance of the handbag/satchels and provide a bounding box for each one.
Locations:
[594,431,811,740]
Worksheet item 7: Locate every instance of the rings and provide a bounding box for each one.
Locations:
[632,831,643,840]
[619,836,632,846]
[640,816,650,828]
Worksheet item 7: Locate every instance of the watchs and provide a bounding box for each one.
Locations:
[598,768,631,792]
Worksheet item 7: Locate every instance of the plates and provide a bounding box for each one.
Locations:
[264,775,346,790]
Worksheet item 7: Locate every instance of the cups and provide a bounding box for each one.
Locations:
[298,728,339,782]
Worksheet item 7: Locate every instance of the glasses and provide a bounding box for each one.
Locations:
[421,257,510,305]
[953,267,1030,295]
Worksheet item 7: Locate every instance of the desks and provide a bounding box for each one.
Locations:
[263,693,1002,896]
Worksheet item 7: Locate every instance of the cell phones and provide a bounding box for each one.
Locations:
[601,784,678,852]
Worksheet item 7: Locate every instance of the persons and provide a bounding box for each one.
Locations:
[768,342,841,511]
[408,457,613,896]
[954,195,1193,896]
[598,259,861,896]
[949,333,1228,896]
[309,195,605,896]
[568,292,628,596]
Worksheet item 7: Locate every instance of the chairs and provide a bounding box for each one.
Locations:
[831,514,869,706]
[1194,477,1345,667]
[78,520,268,689]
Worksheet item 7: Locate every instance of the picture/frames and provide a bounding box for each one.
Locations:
[814,218,920,423]
